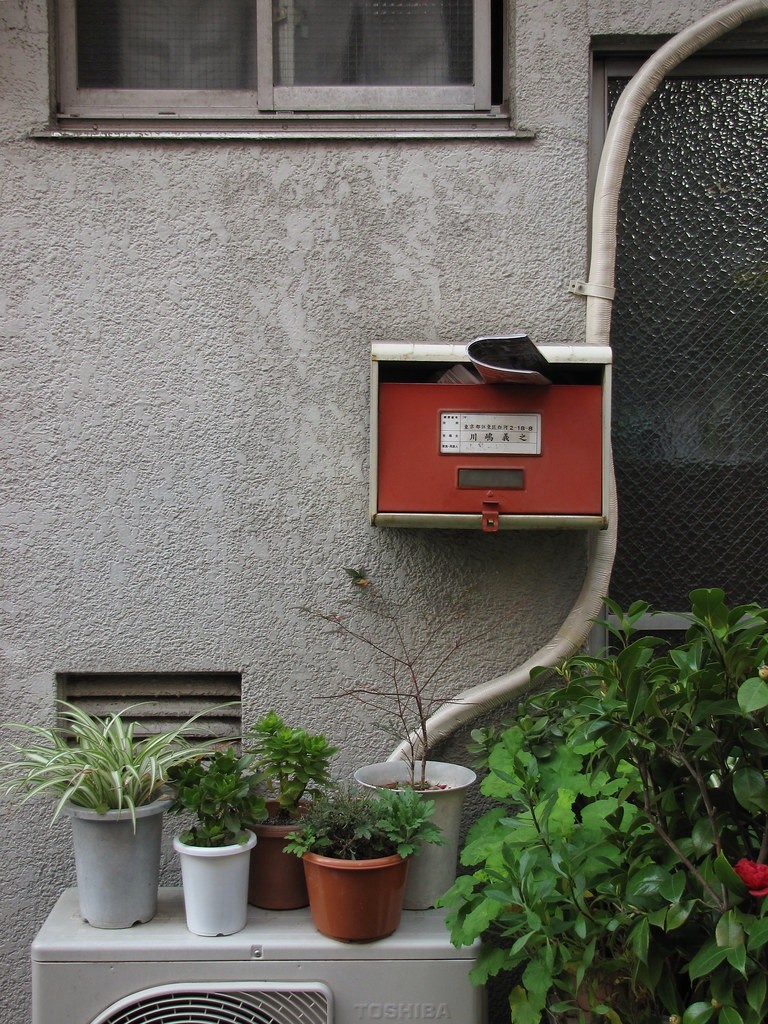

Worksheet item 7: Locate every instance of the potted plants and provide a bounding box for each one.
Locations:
[1,567,476,943]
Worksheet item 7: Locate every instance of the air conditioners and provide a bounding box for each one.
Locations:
[29,887,486,1024]
